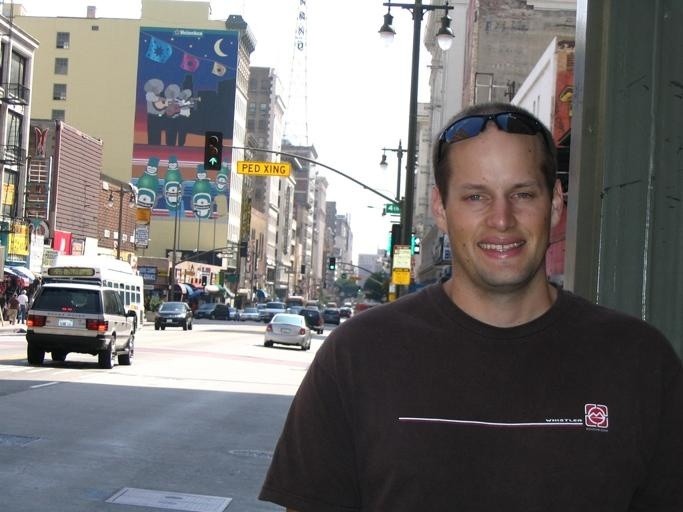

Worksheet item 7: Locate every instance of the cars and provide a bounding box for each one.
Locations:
[153,295,351,350]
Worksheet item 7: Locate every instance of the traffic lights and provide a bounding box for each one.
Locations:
[329,256,335,270]
[204,130,221,169]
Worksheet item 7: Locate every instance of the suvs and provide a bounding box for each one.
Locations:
[24,282,135,369]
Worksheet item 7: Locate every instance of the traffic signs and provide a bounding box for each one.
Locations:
[236,161,289,176]
[385,203,400,214]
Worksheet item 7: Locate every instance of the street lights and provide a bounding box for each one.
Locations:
[172,201,181,251]
[191,209,199,263]
[207,215,215,266]
[377,0,456,298]
[103,184,135,259]
[378,137,420,297]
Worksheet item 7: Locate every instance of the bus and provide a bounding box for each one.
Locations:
[42,255,145,335]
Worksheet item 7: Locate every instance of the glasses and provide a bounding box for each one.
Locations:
[436,111,548,164]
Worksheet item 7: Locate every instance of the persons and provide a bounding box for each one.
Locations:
[6,294,20,326]
[256,101,681,512]
[16,289,29,325]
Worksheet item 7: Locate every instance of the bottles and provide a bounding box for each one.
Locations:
[213,157,229,196]
[163,156,183,212]
[189,164,212,221]
[135,155,160,210]
